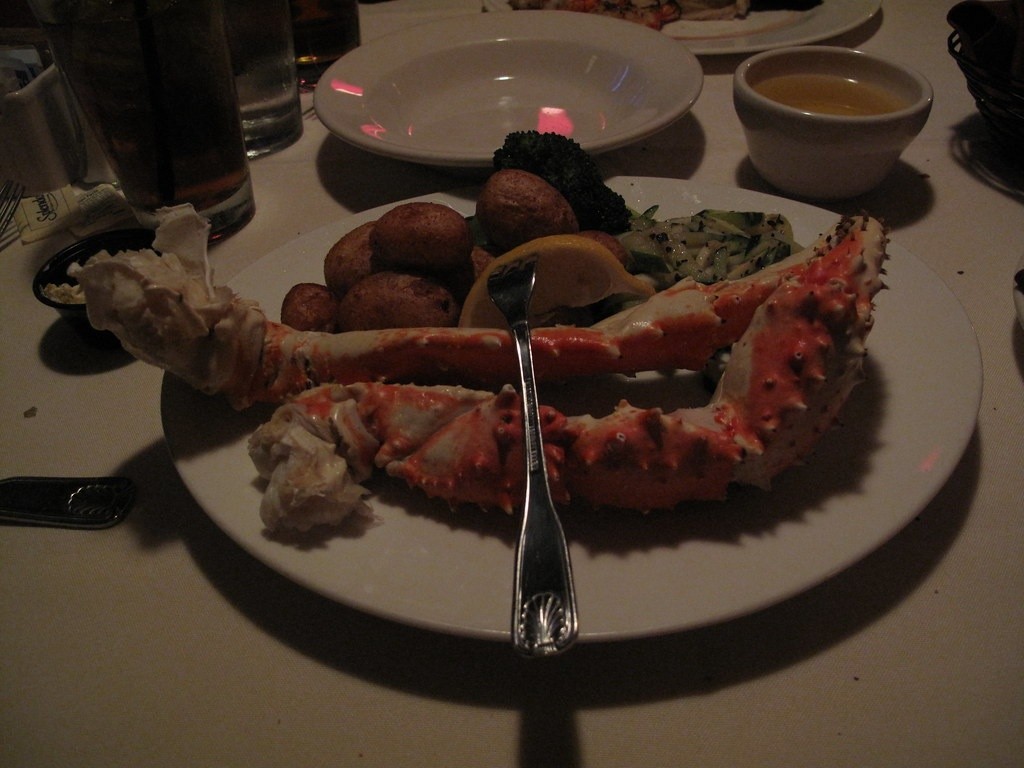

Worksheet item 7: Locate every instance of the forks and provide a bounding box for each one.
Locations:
[0,180,25,238]
[485,253,577,659]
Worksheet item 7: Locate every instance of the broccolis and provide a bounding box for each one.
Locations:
[490,130,634,233]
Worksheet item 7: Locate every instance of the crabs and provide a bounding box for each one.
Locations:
[74,202,891,535]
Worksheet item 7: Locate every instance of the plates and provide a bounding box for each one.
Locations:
[481,0,881,54]
[159,176,982,638]
[311,11,703,167]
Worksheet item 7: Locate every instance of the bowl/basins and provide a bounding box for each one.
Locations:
[734,44,933,197]
[31,228,159,347]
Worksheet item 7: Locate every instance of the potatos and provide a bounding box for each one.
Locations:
[281,169,620,335]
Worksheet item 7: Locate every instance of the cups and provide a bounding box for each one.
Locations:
[221,0,303,162]
[289,1,361,86]
[27,1,254,245]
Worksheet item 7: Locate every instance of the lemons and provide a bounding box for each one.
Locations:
[458,235,654,328]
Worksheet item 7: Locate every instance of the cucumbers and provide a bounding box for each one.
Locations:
[613,204,806,294]
